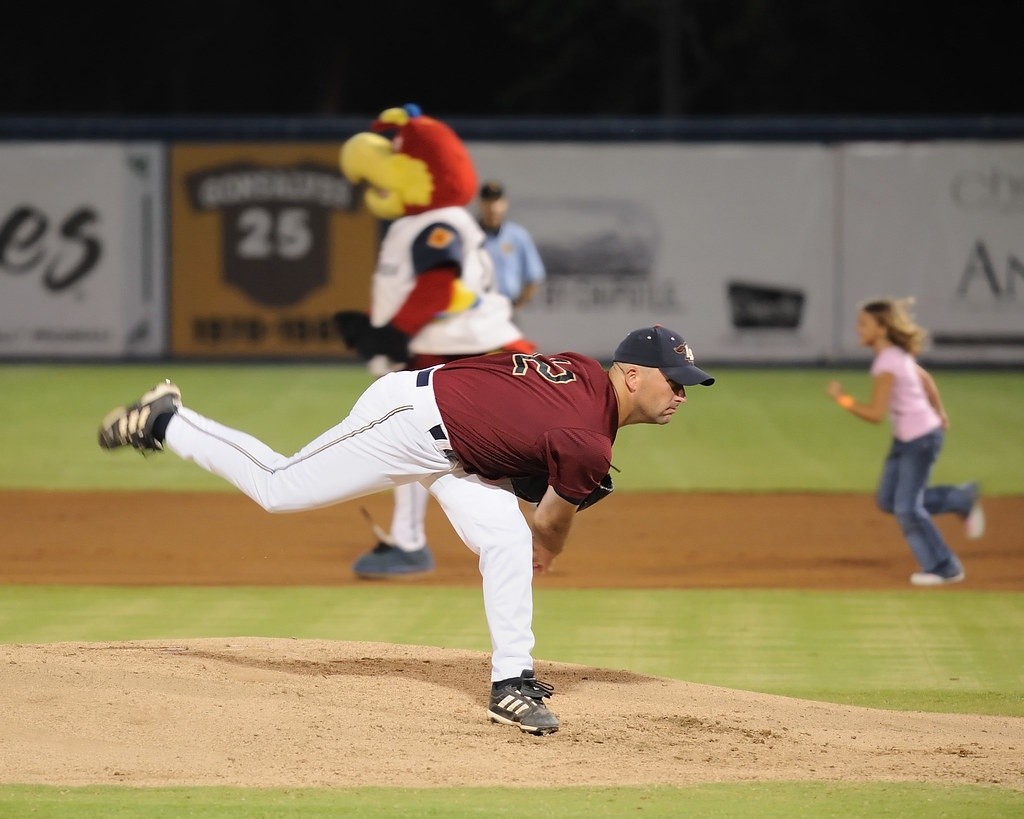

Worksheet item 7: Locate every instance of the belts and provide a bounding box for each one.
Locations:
[417,369,452,455]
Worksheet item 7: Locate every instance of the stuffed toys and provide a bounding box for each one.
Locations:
[339,103,538,580]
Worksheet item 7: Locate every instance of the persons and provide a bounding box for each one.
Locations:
[97,325,716,733]
[473,184,546,308]
[828,301,985,585]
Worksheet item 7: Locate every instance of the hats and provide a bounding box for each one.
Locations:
[614,325,714,387]
[479,183,502,198]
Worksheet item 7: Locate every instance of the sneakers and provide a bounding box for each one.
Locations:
[98,383,182,457]
[486,669,560,733]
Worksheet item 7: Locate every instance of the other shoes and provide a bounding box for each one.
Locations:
[910,572,964,585]
[964,484,985,539]
[354,540,433,574]
